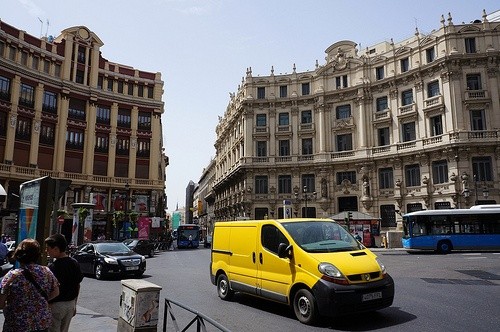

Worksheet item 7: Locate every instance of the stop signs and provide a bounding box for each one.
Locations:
[56,216,65,224]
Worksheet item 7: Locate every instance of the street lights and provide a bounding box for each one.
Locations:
[303,185,309,218]
[474,178,490,205]
[122,182,130,238]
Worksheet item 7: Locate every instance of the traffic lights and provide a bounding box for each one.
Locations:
[347,211,353,221]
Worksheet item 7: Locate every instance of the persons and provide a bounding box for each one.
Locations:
[354,232,362,243]
[0,234,196,332]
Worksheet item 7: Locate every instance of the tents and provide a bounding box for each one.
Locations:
[332,211,383,221]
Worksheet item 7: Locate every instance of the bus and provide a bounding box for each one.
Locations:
[400,204,500,254]
[176,223,202,250]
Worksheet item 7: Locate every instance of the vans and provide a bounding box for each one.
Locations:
[209,216,395,325]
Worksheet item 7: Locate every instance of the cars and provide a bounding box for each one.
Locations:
[71,240,147,280]
[122,238,155,258]
[204,234,212,248]
